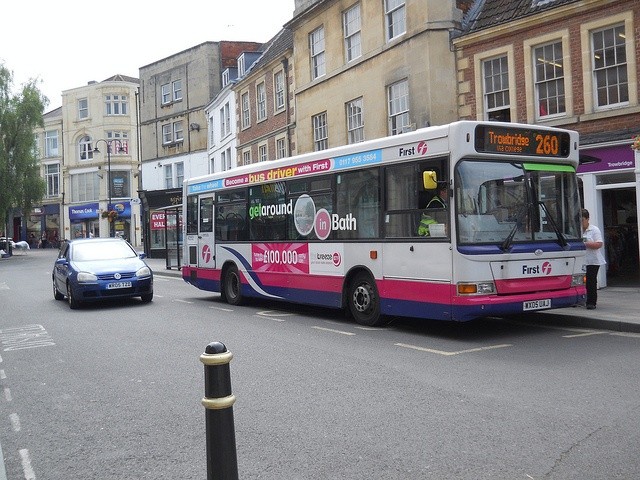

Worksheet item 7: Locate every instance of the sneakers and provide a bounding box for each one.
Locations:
[587,304,596,309]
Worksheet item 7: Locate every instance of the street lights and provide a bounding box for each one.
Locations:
[93,139,124,236]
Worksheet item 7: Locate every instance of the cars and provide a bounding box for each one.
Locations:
[52,237,153,309]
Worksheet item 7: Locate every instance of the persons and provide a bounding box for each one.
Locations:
[42,231,47,247]
[75,229,82,238]
[418,186,450,237]
[115,232,122,237]
[582,208,606,310]
[89,229,95,239]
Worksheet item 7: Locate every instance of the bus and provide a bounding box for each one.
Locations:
[182,121,587,326]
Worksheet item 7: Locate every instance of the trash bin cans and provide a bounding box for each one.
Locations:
[0,237,13,255]
